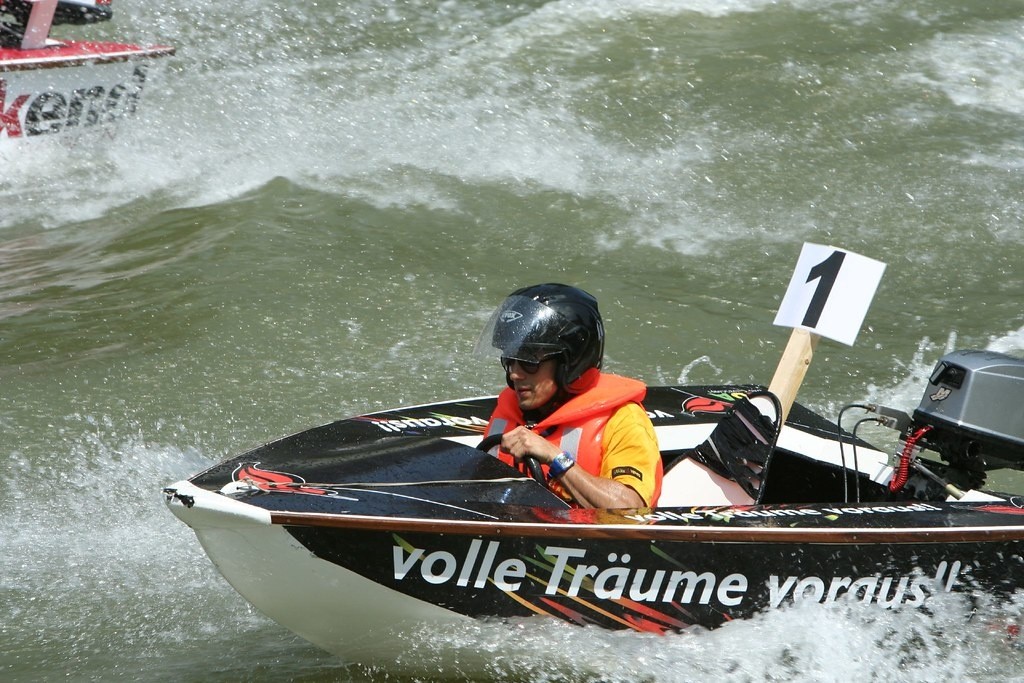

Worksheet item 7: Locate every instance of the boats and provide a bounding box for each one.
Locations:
[0,40,176,144]
[164,348,1024,683]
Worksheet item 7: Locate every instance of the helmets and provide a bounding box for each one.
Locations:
[473,282,605,396]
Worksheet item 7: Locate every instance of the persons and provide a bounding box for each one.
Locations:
[475,284,662,509]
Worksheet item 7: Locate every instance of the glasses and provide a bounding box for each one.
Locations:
[501,350,557,376]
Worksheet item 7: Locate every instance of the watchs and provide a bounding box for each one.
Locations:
[547,451,575,479]
